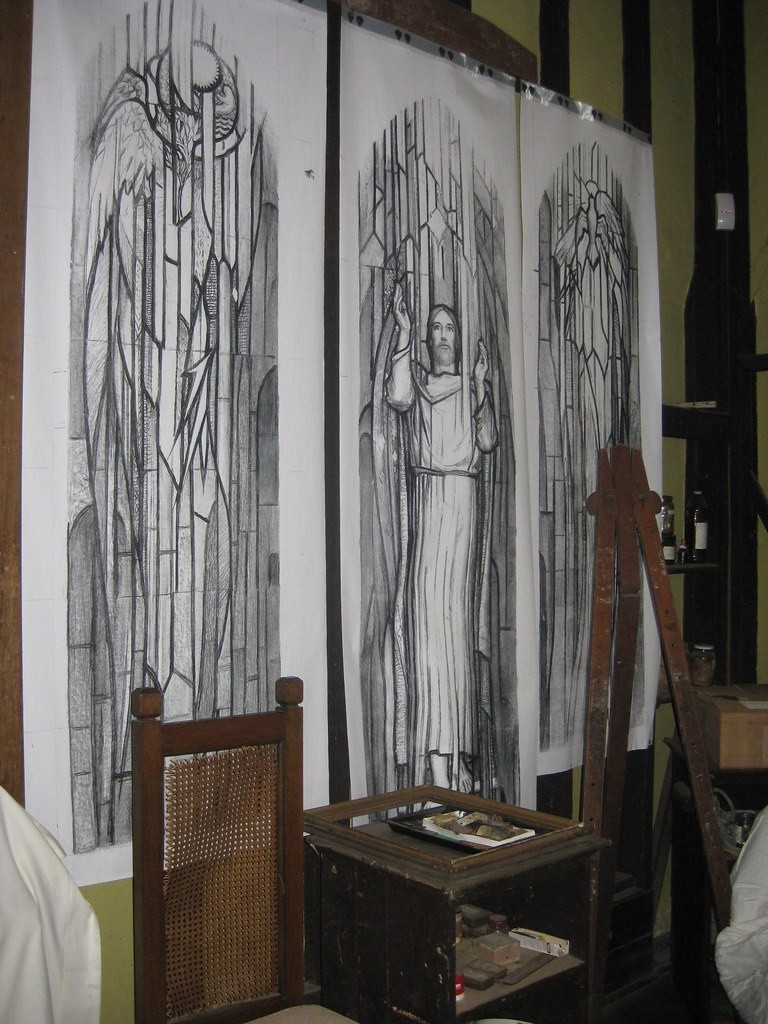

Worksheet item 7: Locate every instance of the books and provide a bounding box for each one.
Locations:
[423,813,535,847]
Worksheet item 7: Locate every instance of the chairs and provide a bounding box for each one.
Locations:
[129,677,358,1024]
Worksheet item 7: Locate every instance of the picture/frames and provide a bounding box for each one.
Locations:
[302,782,598,885]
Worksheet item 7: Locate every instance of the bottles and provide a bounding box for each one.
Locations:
[678,538,686,563]
[456,907,466,1002]
[662,534,676,563]
[691,643,717,685]
[686,490,710,564]
[487,914,508,936]
[662,496,674,535]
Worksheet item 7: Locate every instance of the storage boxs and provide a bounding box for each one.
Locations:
[699,683,768,770]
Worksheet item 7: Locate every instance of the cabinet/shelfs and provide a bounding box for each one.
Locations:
[302,806,613,1024]
[658,403,732,703]
